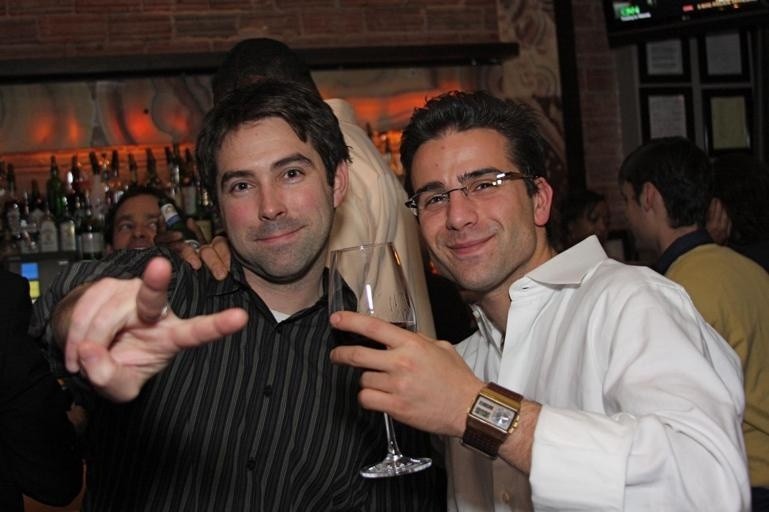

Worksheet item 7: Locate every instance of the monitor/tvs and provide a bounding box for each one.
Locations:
[596,0,769,51]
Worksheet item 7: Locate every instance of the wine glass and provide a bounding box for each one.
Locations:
[326,240,434,479]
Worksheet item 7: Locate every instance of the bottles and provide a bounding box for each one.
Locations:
[364,122,406,179]
[1,141,225,259]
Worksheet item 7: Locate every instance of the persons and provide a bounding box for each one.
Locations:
[557,184,734,276]
[167,87,753,511]
[424,272,477,344]
[103,189,185,253]
[153,37,438,339]
[617,133,768,512]
[28,75,448,512]
[2,274,88,512]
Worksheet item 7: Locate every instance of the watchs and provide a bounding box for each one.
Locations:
[460,383,524,460]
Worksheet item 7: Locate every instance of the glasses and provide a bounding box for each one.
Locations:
[405,169,534,216]
